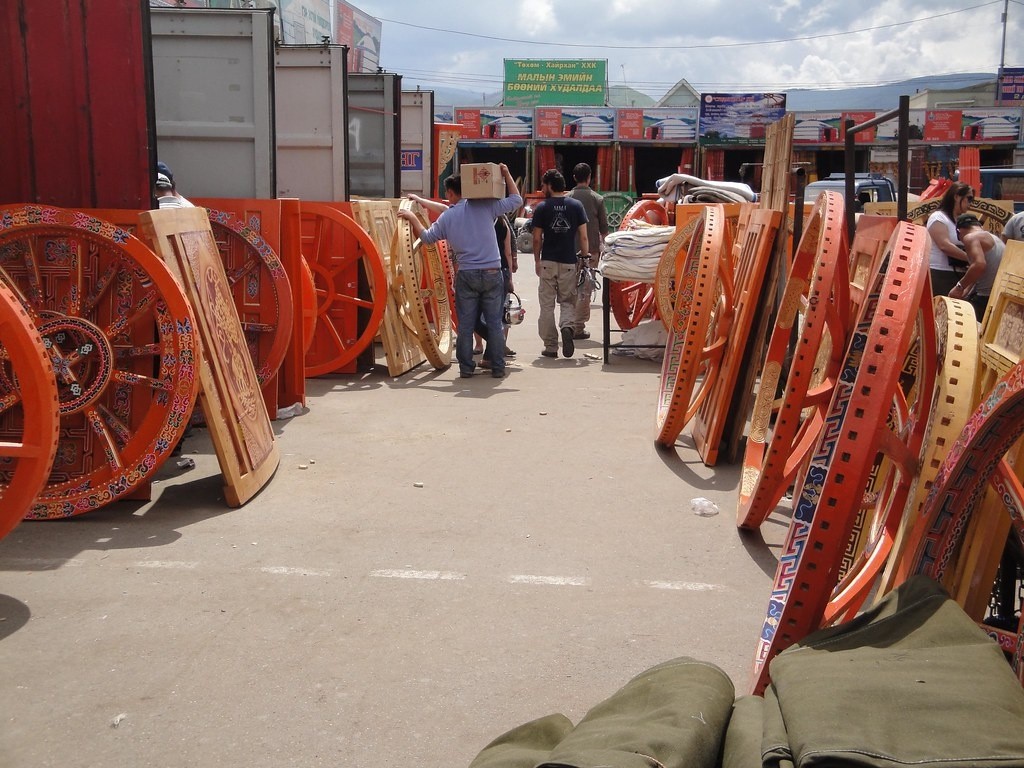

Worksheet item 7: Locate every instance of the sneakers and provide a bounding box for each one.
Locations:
[492,364,505,378]
[460,372,474,378]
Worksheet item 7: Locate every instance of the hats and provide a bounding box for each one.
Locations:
[157,161,173,178]
[156,173,172,188]
[956,213,981,241]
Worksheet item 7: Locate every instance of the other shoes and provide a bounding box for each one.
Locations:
[573,330,590,339]
[562,327,574,358]
[504,346,516,355]
[477,359,492,367]
[541,349,558,358]
[473,345,483,355]
[169,449,181,457]
[184,430,192,437]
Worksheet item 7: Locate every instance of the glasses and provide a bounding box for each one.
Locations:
[959,194,974,204]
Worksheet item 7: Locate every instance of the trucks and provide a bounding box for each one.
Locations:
[791,168,897,214]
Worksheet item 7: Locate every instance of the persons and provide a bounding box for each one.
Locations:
[155,161,198,209]
[396,162,523,379]
[998,211,1024,246]
[531,169,589,359]
[925,181,969,297]
[947,212,1006,323]
[563,163,609,339]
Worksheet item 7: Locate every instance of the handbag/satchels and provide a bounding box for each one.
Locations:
[502,291,525,325]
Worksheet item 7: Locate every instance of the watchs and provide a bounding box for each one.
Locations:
[955,280,965,292]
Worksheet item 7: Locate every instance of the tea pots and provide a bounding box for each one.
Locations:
[504,291,524,325]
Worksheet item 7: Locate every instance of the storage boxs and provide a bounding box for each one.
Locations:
[460,163,506,200]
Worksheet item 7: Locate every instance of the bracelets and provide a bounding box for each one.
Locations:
[512,256,517,259]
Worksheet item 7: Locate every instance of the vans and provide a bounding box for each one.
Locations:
[952,167,1024,215]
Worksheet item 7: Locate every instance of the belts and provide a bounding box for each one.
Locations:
[464,270,498,273]
